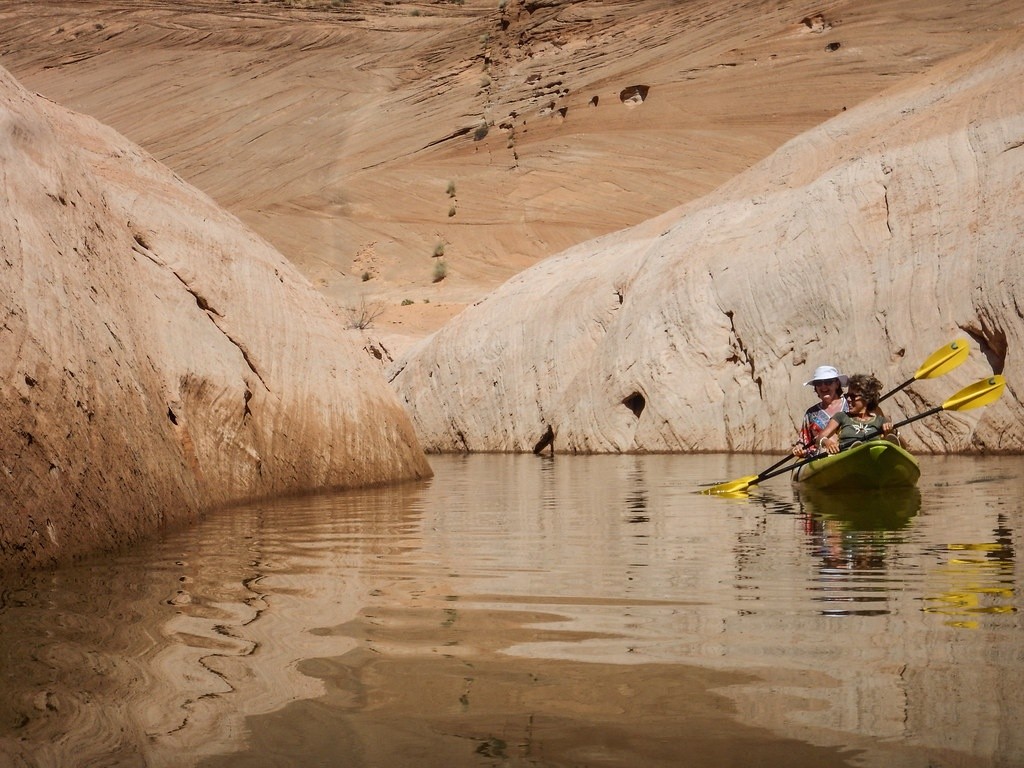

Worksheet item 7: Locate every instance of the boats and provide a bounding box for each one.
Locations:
[790,433,922,488]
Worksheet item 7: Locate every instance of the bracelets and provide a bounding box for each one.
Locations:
[820,437,827,446]
[895,429,899,436]
[799,442,805,446]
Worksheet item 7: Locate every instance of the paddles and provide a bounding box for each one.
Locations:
[726,338,970,483]
[704,374,1006,490]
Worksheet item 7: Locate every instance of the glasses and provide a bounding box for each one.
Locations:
[813,380,838,386]
[844,394,862,400]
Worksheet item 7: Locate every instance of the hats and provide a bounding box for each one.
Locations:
[804,366,848,390]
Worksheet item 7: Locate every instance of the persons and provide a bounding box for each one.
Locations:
[792,366,849,459]
[816,375,907,455]
